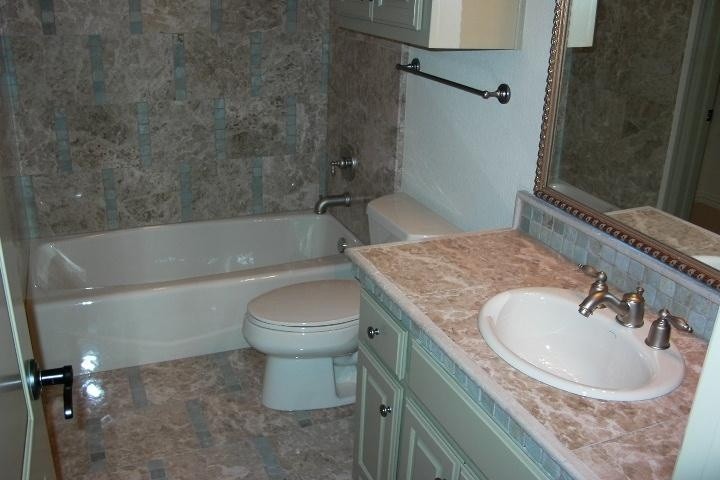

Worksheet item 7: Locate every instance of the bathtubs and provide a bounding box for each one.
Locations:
[24,211,366,375]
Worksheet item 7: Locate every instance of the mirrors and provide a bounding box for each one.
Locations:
[533,1,720,295]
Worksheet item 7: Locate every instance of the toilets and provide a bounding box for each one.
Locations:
[240,191,466,412]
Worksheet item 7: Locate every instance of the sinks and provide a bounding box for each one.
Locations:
[477,286,685,401]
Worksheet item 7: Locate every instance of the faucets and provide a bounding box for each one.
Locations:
[352,193,376,206]
[313,191,352,215]
[577,286,645,329]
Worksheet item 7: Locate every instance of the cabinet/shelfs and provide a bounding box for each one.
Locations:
[335,1,423,32]
[352,290,547,479]
